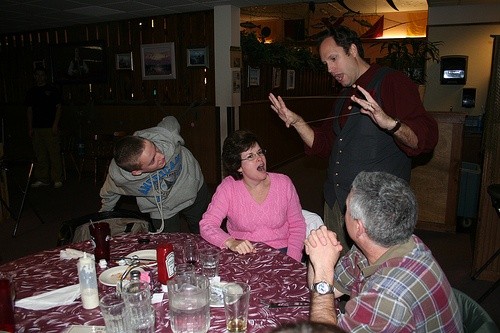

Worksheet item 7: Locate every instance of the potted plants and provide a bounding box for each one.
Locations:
[370,38,443,103]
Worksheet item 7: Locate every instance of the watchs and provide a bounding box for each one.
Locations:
[311,281,334,295]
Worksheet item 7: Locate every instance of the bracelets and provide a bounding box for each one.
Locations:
[387,121,401,133]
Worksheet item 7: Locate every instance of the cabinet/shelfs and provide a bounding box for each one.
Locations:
[411,111,469,233]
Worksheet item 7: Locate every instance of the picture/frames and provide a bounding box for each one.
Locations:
[115,52,133,70]
[186,47,209,67]
[140,42,176,80]
[286,69,295,89]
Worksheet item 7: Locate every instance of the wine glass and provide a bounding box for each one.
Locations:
[183,245,199,273]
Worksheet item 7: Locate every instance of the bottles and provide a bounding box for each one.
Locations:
[157,244,176,284]
[77,252,100,310]
[0,278,16,333]
[128,270,142,291]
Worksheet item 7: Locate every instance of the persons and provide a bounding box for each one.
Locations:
[199,130,307,263]
[304,169,464,333]
[25,67,65,187]
[268,27,438,269]
[97,116,229,233]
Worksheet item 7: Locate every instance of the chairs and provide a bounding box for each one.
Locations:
[79,127,110,184]
[59,209,150,244]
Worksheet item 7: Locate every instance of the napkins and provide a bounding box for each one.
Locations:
[14,283,82,310]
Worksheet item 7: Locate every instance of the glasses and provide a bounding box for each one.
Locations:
[240,148,267,161]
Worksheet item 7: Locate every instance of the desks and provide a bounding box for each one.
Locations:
[0,232,316,333]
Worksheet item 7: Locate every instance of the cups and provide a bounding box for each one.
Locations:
[100,279,156,333]
[167,274,211,333]
[223,281,251,333]
[89,222,111,262]
[199,248,220,277]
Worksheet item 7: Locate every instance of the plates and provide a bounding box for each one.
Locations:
[209,282,243,308]
[125,249,157,267]
[98,265,130,287]
